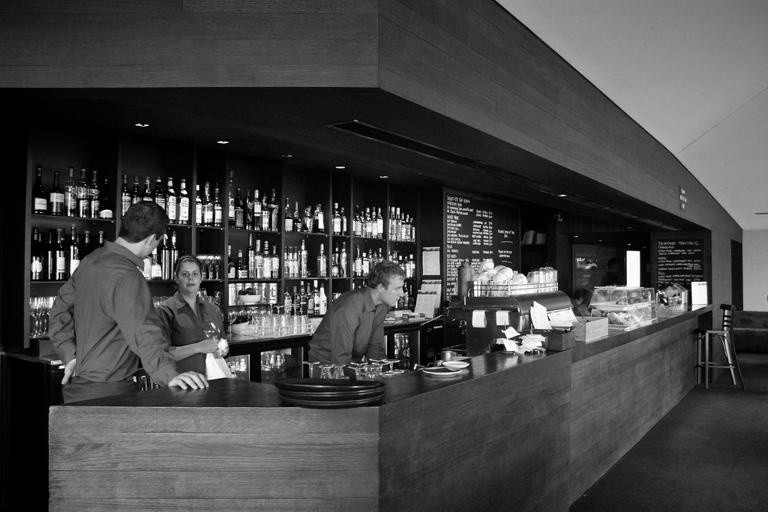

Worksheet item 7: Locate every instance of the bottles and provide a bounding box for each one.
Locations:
[389,248,417,276]
[229,282,278,303]
[331,241,349,278]
[131,375,159,392]
[457,261,472,297]
[354,243,385,276]
[388,205,415,242]
[316,243,328,278]
[228,233,279,278]
[228,170,279,230]
[527,266,558,292]
[356,202,383,239]
[121,167,224,225]
[332,199,349,236]
[391,281,415,309]
[123,230,180,282]
[284,281,331,317]
[30,227,116,279]
[394,335,411,358]
[281,194,325,232]
[30,296,56,338]
[31,167,112,219]
[228,349,288,378]
[284,235,311,278]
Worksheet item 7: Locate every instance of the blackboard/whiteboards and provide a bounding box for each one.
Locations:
[442,188,522,302]
[650,229,712,305]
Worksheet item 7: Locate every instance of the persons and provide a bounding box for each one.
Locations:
[307,260,405,379]
[48,201,210,391]
[156,256,236,381]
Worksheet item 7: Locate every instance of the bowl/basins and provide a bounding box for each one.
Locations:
[476,258,526,298]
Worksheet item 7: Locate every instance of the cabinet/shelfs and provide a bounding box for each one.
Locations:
[213,310,438,382]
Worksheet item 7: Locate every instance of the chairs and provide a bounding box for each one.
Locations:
[691,304,748,390]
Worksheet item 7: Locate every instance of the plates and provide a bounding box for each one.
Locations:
[442,361,470,369]
[423,367,461,376]
[273,378,387,408]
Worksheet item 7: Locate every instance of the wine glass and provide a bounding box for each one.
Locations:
[300,359,402,379]
[201,321,221,358]
[228,303,313,336]
[193,254,222,281]
[197,287,223,306]
[151,296,169,307]
